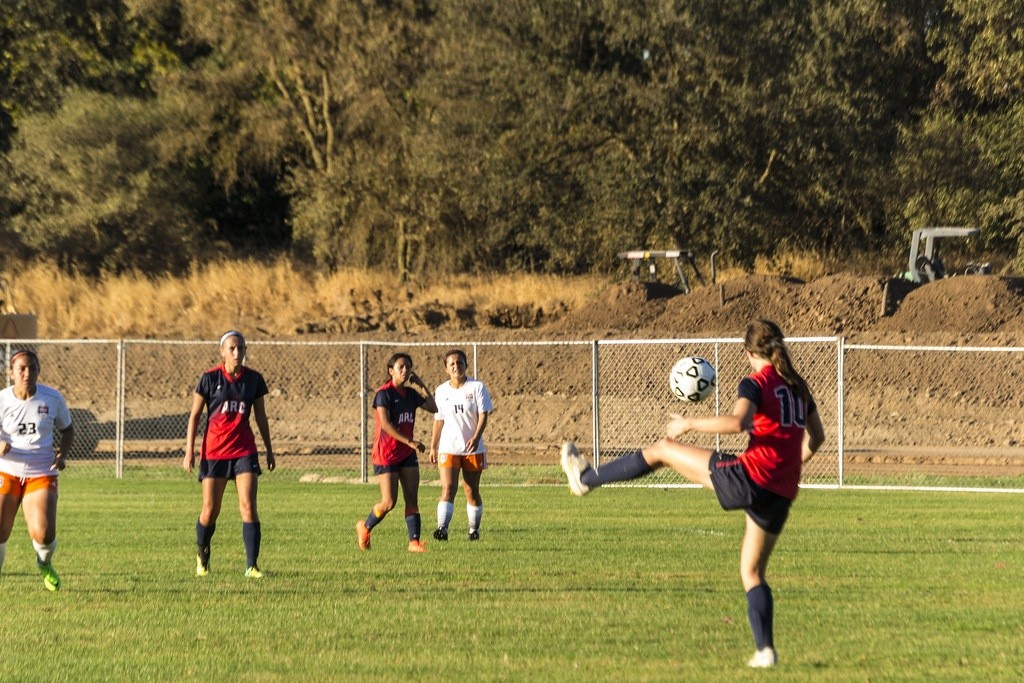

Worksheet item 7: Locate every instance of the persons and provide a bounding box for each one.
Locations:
[0,349,75,591]
[183,330,276,578]
[355,352,439,552]
[561,317,826,670]
[428,349,493,541]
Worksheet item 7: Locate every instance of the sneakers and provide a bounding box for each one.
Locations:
[559,442,591,497]
[408,540,428,552]
[245,565,264,579]
[748,646,778,668]
[468,531,480,542]
[196,545,211,576]
[356,520,372,551]
[35,554,61,592]
[433,523,448,542]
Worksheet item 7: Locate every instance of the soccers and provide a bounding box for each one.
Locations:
[669,357,717,403]
